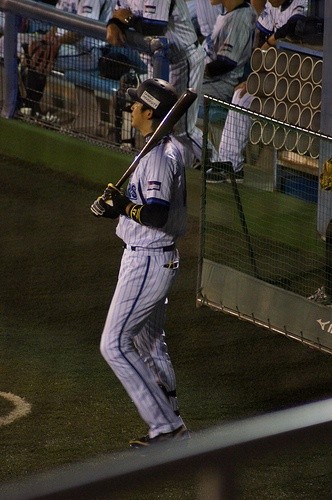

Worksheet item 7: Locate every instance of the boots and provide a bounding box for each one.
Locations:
[18,64,36,100]
[17,68,46,117]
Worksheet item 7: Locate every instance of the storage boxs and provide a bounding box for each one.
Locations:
[276,165,318,204]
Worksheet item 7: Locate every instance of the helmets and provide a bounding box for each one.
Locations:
[127,78,180,113]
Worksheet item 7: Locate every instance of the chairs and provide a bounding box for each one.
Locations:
[0,0,252,101]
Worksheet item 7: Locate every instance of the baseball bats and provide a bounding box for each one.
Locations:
[21,40,48,116]
[89,90,198,217]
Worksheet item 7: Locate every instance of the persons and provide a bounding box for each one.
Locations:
[206,0,326,183]
[183,0,226,43]
[91,79,190,450]
[1,0,103,120]
[195,0,257,100]
[104,0,222,174]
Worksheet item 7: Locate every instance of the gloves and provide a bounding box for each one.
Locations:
[101,182,130,215]
[89,196,121,220]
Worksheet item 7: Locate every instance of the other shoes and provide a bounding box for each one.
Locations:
[129,426,185,447]
[201,164,244,184]
[308,288,332,309]
